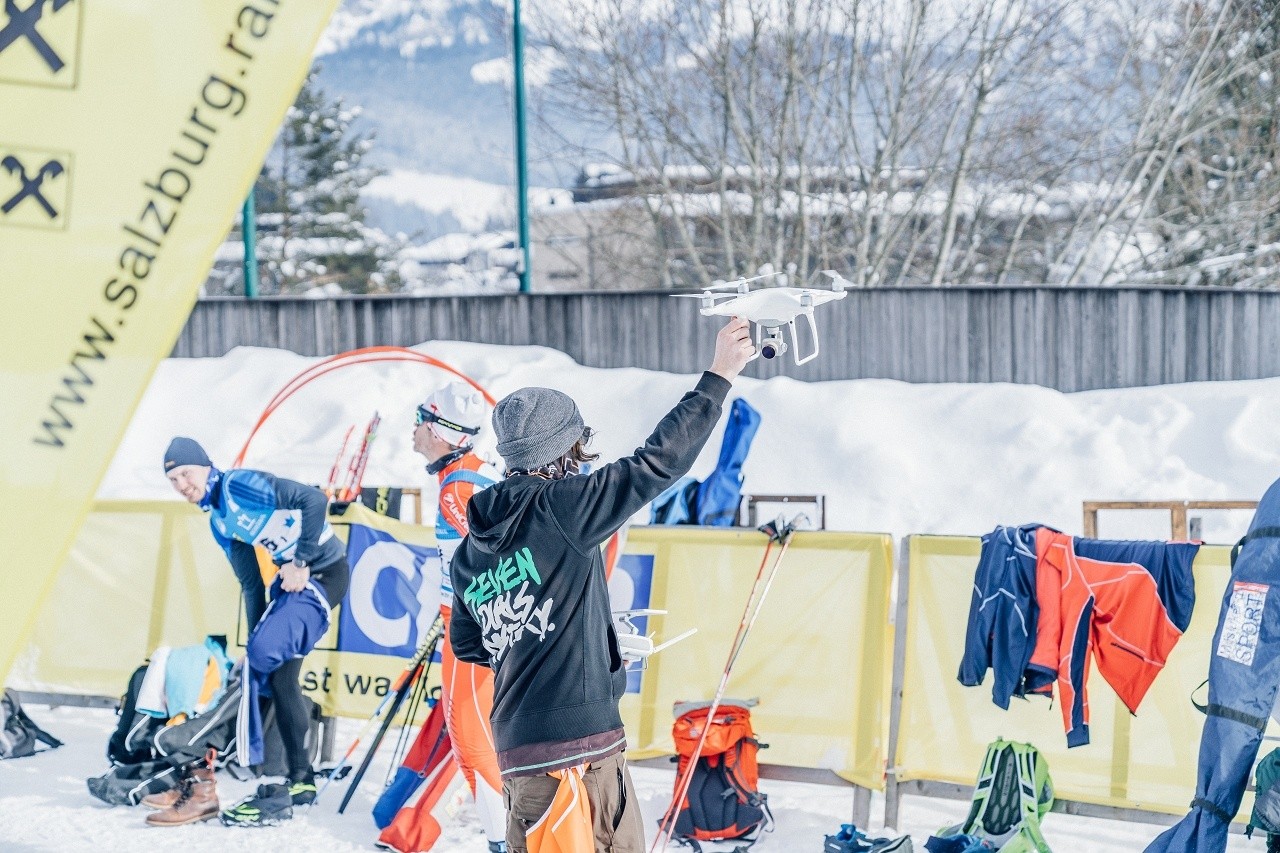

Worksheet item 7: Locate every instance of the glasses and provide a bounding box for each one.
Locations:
[415,405,435,425]
[575,427,591,445]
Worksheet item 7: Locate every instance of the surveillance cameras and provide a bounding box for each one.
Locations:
[762,337,788,359]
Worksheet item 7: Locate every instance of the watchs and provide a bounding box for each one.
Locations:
[292,555,307,569]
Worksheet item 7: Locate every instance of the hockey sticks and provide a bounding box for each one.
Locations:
[649,511,813,853]
[311,612,442,817]
[324,410,383,502]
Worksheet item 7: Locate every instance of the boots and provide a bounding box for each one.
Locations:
[145,779,220,825]
[140,768,216,808]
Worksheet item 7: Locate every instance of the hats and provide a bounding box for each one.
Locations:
[491,386,584,470]
[421,382,484,447]
[164,437,214,473]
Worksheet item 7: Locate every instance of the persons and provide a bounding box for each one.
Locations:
[165,437,351,830]
[411,317,755,853]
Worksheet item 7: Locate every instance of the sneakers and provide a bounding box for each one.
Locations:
[283,781,316,806]
[824,824,875,853]
[868,835,913,853]
[218,784,294,822]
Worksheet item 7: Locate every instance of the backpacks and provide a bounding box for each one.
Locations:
[660,697,776,853]
[87,659,326,808]
[937,736,1055,853]
[0,687,65,759]
